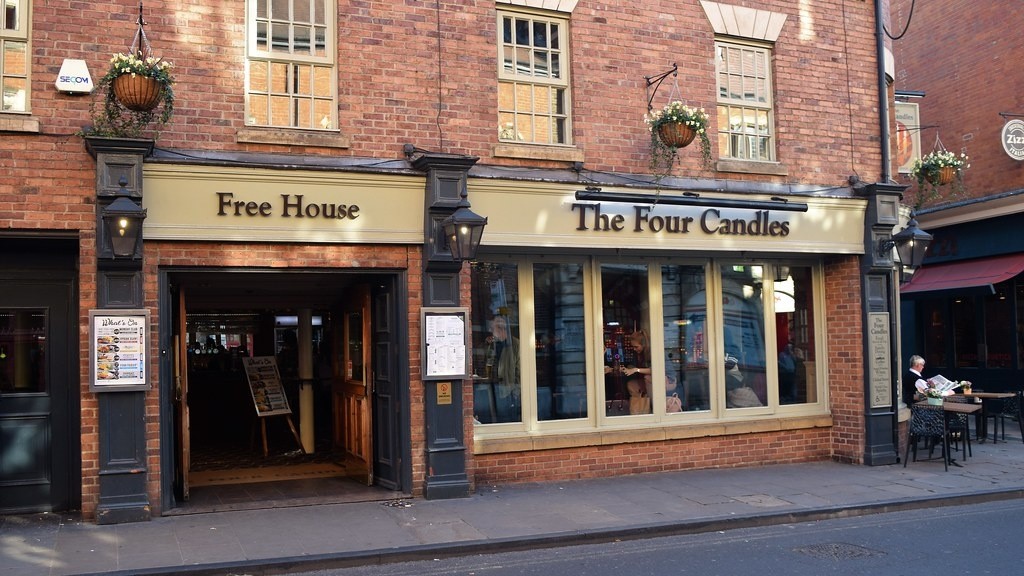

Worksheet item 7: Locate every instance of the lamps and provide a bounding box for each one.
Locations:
[440,181,489,265]
[887,211,936,289]
[97,171,152,259]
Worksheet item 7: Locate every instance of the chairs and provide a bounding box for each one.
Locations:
[903,400,982,468]
[982,390,1024,445]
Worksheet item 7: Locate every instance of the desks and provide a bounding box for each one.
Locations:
[912,391,1016,466]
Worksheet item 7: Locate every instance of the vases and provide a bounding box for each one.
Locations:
[112,73,165,113]
[654,122,698,148]
[921,165,959,188]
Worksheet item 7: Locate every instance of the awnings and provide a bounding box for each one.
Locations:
[900,252,1024,296]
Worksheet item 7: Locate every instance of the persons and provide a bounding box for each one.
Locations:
[620,330,679,399]
[485,316,521,417]
[903,354,955,407]
[778,342,807,405]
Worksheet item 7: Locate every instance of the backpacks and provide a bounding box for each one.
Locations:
[666,393,682,413]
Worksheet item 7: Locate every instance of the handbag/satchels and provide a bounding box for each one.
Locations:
[629,392,651,415]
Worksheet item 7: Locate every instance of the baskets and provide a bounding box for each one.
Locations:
[923,163,958,186]
[658,120,696,148]
[114,74,165,113]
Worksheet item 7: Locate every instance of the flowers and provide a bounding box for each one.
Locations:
[913,130,976,212]
[78,50,175,138]
[644,100,710,208]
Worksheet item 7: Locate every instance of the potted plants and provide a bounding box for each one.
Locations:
[927,388,945,406]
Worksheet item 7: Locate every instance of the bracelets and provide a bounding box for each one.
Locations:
[638,368,640,373]
